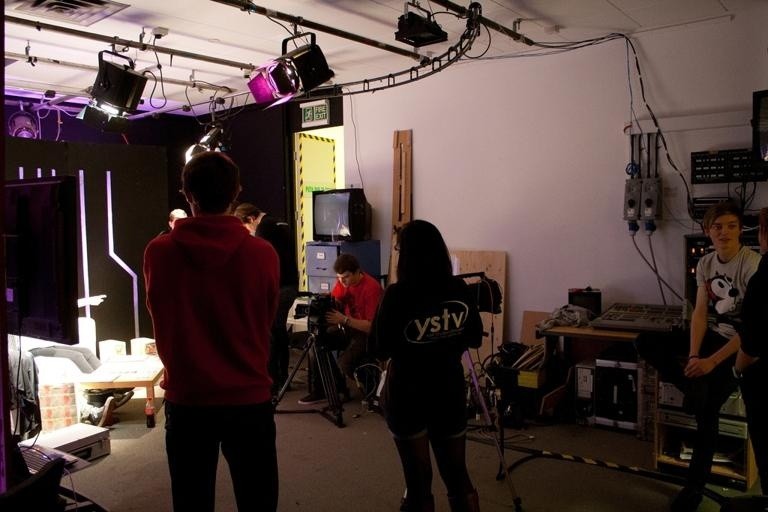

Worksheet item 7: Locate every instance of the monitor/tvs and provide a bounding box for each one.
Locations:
[0,175,79,343]
[312,187,374,242]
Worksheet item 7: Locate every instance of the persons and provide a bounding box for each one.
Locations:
[142,151,280,512]
[158,209,188,236]
[299,255,383,406]
[235,204,298,393]
[732,205,767,498]
[635,200,760,510]
[377,220,483,510]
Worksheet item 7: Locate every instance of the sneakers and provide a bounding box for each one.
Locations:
[664,485,703,512]
[298,392,328,405]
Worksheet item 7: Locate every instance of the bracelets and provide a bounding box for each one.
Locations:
[344,315,350,324]
[687,355,698,360]
[732,367,743,380]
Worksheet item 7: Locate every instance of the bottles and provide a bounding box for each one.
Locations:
[144,397,156,428]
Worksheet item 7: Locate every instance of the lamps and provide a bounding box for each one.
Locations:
[8,111,38,139]
[248,70,293,110]
[271,31,335,96]
[395,3,448,47]
[198,100,226,152]
[91,51,147,114]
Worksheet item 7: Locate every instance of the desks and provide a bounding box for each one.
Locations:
[83,357,167,428]
[537,323,641,421]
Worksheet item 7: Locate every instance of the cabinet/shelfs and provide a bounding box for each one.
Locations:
[306,241,379,293]
[652,367,758,491]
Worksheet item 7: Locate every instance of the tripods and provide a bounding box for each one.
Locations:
[277,338,352,428]
